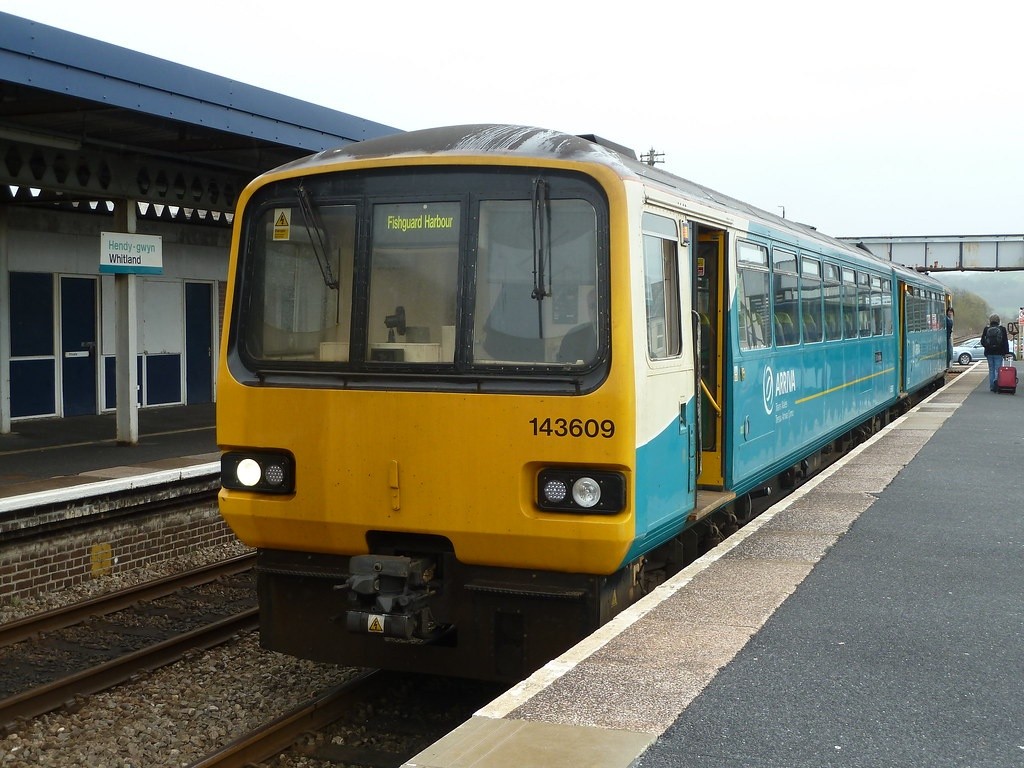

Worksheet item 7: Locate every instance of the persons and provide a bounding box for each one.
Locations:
[981,314,1009,393]
[946,307,954,368]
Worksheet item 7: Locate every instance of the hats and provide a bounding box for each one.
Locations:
[989,315,1000,322]
[947,306,955,312]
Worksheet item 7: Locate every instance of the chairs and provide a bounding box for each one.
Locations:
[843,313,853,338]
[777,312,797,345]
[826,313,836,340]
[802,313,820,342]
[812,313,831,340]
[788,312,809,343]
[761,313,784,346]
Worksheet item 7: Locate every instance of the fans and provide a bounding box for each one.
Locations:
[384,305,406,343]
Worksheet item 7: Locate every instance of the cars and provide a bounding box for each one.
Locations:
[952,336,1017,365]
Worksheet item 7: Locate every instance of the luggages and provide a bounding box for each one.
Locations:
[996,356,1019,395]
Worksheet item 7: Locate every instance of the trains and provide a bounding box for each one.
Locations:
[213,126,953,652]
